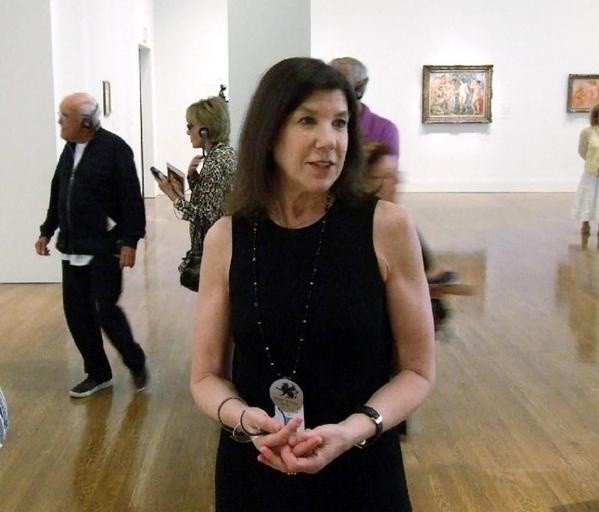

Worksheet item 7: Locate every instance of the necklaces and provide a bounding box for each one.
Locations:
[251,201,334,413]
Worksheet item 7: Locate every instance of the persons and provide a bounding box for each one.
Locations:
[358,142,451,319]
[35,93,151,398]
[329,57,399,204]
[190,52,435,512]
[435,75,481,114]
[150,96,237,290]
[577,104,599,241]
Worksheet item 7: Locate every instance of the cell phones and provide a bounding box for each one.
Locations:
[151,167,167,181]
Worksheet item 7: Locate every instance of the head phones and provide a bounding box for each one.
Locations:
[81,103,99,129]
[199,128,208,138]
[354,77,368,99]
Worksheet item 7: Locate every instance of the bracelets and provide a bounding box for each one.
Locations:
[219,397,246,441]
[354,406,384,451]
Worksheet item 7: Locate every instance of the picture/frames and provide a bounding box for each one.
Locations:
[103,80,112,116]
[423,64,494,125]
[567,73,599,113]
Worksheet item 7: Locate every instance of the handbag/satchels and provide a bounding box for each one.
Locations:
[179,250,201,292]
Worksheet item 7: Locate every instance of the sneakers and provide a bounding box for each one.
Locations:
[69,378,113,398]
[133,368,147,391]
